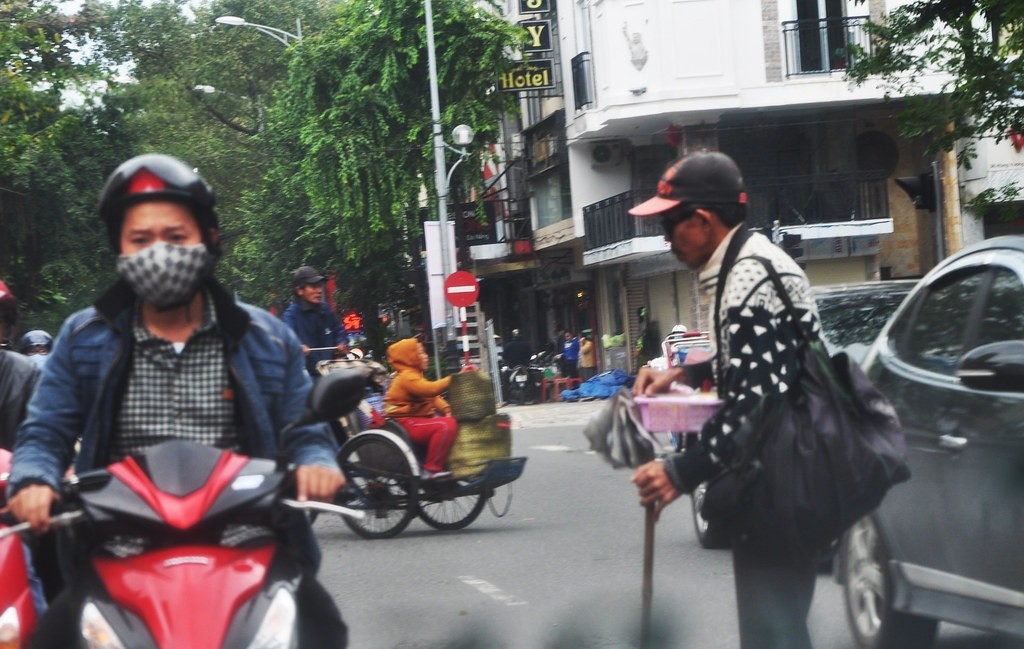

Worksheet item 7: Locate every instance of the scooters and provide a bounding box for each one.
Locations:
[0,438,366,649]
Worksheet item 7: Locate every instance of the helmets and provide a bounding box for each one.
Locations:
[20,330,53,352]
[97,153,217,217]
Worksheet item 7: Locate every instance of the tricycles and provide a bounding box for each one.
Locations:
[307,347,528,539]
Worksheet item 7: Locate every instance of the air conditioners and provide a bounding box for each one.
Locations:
[587,139,622,168]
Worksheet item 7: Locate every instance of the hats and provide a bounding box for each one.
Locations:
[627,147,747,216]
[293,266,329,288]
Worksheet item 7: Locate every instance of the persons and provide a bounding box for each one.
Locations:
[561,331,595,381]
[383,340,479,477]
[496,330,536,405]
[628,151,827,648]
[0,281,54,449]
[284,269,349,373]
[4,153,348,649]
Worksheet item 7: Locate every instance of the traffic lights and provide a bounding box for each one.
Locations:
[894,173,938,213]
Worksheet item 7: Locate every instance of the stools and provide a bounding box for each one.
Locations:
[542,377,583,401]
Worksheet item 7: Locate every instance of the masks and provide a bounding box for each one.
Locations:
[115,241,212,312]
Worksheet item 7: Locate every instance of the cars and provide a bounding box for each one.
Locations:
[831,235,1024,649]
[688,280,920,550]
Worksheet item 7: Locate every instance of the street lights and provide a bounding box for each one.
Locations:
[214,15,304,50]
[193,83,266,138]
[423,0,474,373]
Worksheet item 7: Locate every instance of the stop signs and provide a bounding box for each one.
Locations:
[444,270,479,307]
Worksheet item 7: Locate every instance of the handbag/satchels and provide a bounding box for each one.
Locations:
[702,353,914,549]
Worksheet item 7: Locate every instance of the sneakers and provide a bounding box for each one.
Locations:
[421,467,453,480]
